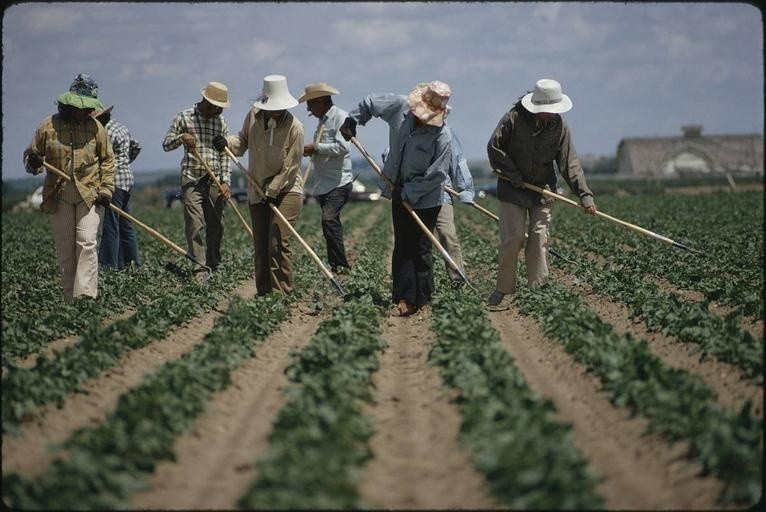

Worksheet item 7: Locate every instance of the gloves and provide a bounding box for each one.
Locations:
[28,151,43,168]
[340,117,356,140]
[391,187,402,203]
[262,196,277,208]
[98,192,112,207]
[213,135,227,152]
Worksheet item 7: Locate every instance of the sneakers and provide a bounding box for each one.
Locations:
[388,300,417,318]
[489,290,504,305]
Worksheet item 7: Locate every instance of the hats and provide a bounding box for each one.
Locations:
[299,83,340,103]
[253,76,298,111]
[201,82,231,107]
[55,73,113,120]
[522,80,573,114]
[406,82,451,128]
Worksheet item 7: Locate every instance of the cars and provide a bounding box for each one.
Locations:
[163,181,248,210]
[27,185,43,210]
[477,174,566,201]
[302,180,381,204]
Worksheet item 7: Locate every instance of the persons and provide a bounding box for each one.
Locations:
[212,73,304,301]
[161,80,233,273]
[485,77,596,297]
[380,124,476,289]
[90,102,142,270]
[295,81,355,273]
[338,78,454,318]
[20,71,115,306]
